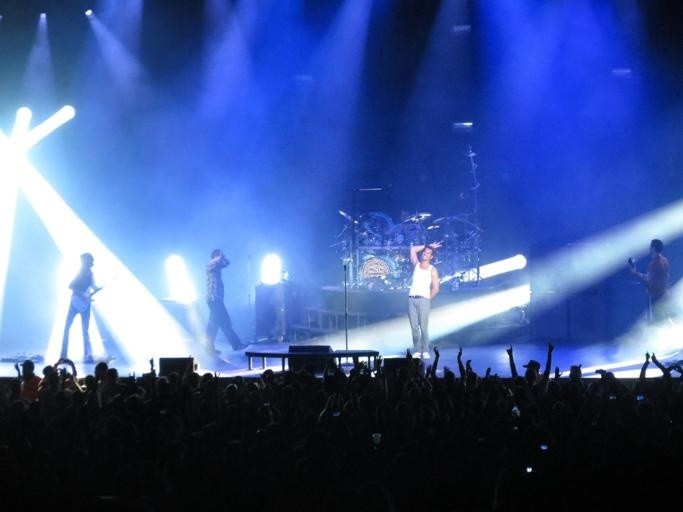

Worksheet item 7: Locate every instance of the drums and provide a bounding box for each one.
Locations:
[358,255,396,280]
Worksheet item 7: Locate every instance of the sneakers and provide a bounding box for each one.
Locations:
[411,352,430,359]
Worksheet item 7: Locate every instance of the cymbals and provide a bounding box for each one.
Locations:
[404,213,432,222]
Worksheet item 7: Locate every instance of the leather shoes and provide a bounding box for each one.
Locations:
[233,345,250,351]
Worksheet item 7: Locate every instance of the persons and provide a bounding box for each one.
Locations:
[204,248,251,355]
[60,252,95,364]
[0,338,683,512]
[631,239,672,369]
[406,240,442,360]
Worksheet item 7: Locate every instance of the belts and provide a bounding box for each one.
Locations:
[408,295,423,299]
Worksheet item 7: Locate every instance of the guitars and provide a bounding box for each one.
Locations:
[71,287,101,313]
[628,257,647,286]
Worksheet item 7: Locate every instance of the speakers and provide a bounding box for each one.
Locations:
[289,345,337,374]
[383,357,426,378]
[159,357,194,377]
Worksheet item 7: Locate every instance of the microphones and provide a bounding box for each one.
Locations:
[338,209,359,226]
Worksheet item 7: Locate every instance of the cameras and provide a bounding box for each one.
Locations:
[525,467,533,474]
[541,444,548,450]
[332,411,340,416]
[635,394,645,401]
